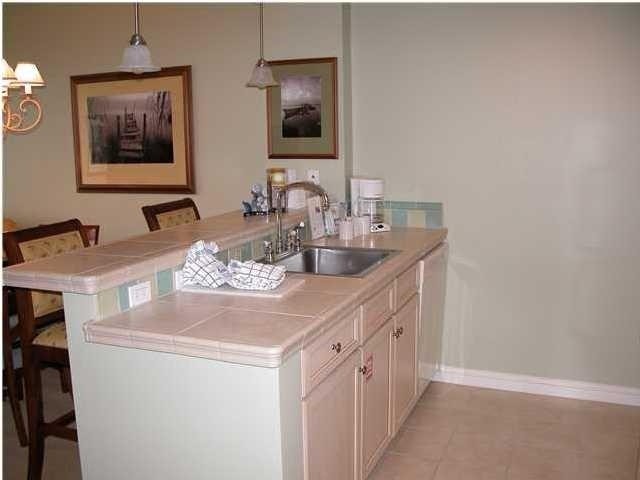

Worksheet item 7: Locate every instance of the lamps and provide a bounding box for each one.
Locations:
[117,3,162,76]
[3,57,48,138]
[245,3,282,92]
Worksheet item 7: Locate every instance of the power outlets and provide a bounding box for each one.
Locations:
[127,279,154,310]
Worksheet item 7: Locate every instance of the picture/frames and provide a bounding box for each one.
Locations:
[68,63,197,197]
[264,56,341,161]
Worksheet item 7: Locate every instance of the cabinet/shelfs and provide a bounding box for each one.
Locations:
[302,306,362,479]
[363,263,419,480]
[418,241,448,392]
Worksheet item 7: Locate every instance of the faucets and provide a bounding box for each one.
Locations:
[275,182,328,254]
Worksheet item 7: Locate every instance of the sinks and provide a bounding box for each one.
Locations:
[253,244,402,279]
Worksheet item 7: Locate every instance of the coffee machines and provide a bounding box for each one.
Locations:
[351,178,391,233]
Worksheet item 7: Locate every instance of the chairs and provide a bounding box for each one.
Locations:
[4,216,102,479]
[140,197,202,232]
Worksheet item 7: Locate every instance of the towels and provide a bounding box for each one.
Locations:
[224,258,288,291]
[182,240,233,289]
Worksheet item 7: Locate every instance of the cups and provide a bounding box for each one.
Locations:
[339,223,353,240]
[357,217,369,235]
